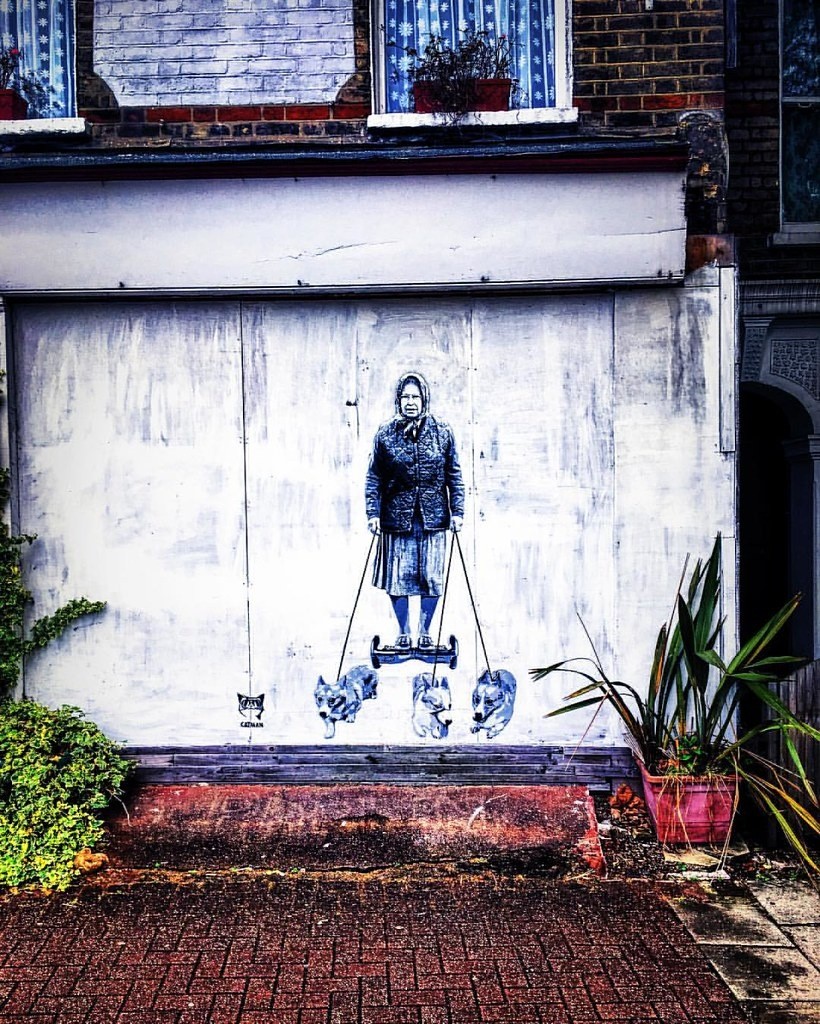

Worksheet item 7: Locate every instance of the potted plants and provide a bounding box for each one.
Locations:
[526,526,820,895]
[380,24,463,112]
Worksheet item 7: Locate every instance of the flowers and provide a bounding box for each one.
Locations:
[0,44,26,87]
[464,29,525,78]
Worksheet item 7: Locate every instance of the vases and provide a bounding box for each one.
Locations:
[467,77,512,114]
[0,89,15,120]
